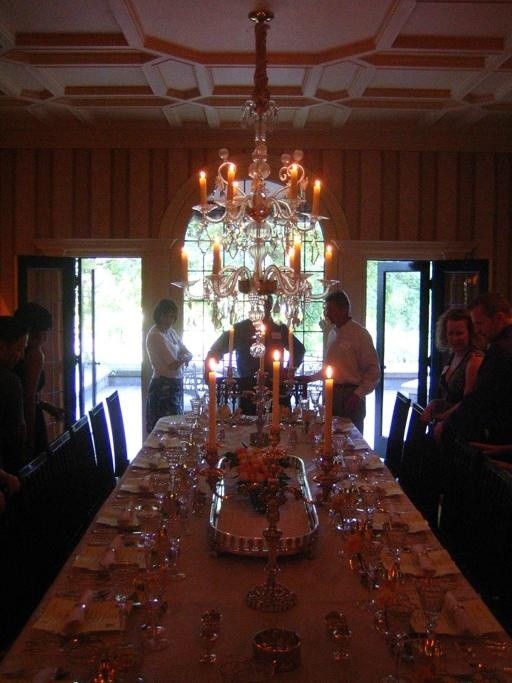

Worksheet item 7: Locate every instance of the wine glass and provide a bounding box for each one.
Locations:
[59,386,452,683]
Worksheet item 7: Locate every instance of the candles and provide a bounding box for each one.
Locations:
[209,357,216,446]
[273,348,280,429]
[259,323,265,368]
[229,324,234,366]
[325,366,333,454]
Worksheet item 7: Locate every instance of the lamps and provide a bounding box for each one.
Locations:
[170,8,341,358]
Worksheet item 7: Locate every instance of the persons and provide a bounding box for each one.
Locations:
[1,314,30,474]
[0,468,21,515]
[416,306,485,424]
[203,289,307,416]
[146,295,193,439]
[465,440,511,475]
[292,288,382,438]
[432,290,512,458]
[12,299,71,459]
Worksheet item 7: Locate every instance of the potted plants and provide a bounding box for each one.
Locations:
[289,324,294,368]
